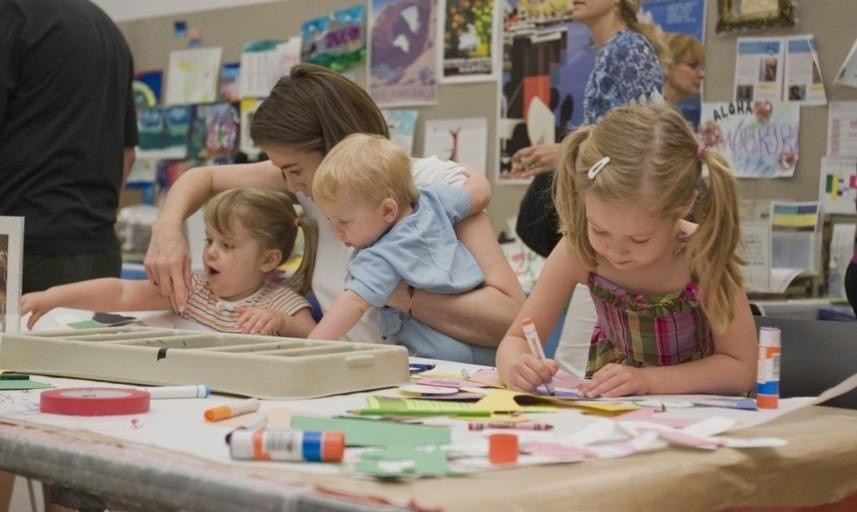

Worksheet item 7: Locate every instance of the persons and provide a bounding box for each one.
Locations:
[0,1,140,294]
[495,108,757,397]
[658,33,706,107]
[509,0,673,180]
[145,65,527,350]
[303,132,497,367]
[20,186,319,339]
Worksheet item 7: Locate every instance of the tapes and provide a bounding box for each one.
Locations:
[39,387,150,415]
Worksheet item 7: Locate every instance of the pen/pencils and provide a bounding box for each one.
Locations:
[345,409,491,417]
[469,422,554,431]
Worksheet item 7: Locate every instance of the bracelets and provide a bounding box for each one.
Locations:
[407,285,414,320]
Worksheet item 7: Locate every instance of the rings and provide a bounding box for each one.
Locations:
[151,279,160,288]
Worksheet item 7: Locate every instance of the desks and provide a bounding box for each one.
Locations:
[0,372,857,511]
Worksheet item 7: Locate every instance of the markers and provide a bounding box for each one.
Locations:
[521,318,556,396]
[203,398,260,422]
[144,385,209,399]
